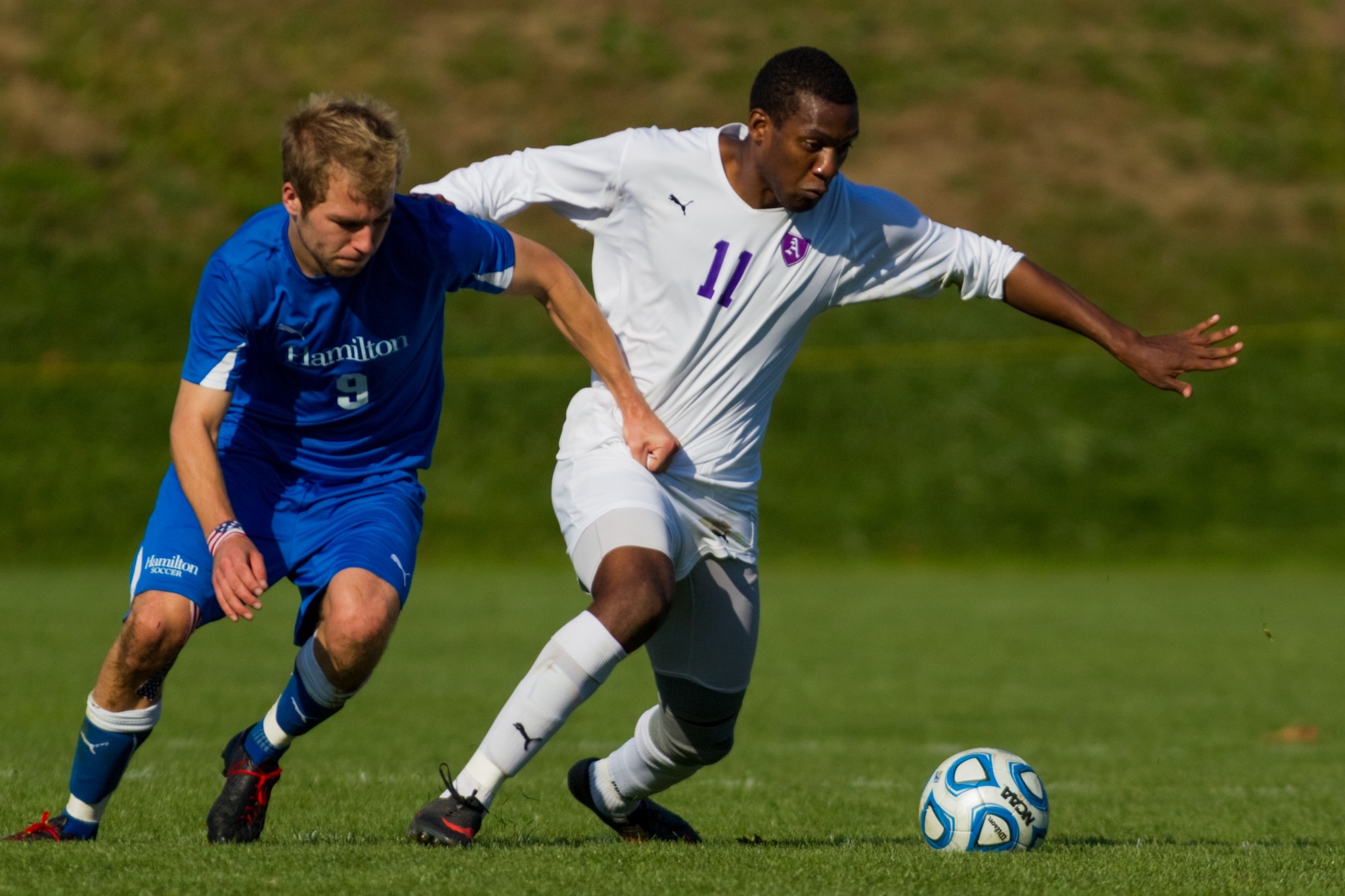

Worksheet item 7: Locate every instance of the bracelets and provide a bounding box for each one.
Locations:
[206,519,246,556]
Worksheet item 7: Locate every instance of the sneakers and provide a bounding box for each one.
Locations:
[406,762,488,849]
[206,722,282,845]
[568,757,702,845]
[4,812,99,843]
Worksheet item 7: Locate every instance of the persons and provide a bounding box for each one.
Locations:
[405,45,1244,849]
[0,89,680,849]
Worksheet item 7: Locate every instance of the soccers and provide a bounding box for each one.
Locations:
[918,747,1051,852]
